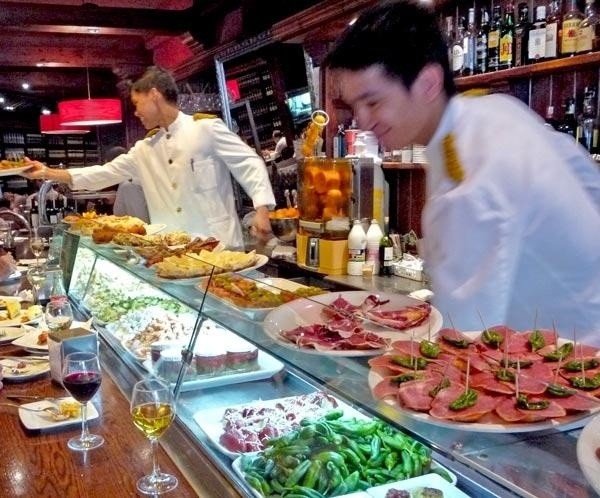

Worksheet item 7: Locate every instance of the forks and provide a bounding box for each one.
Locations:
[2,392,59,414]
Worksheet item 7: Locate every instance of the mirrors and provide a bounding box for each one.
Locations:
[213,1,377,221]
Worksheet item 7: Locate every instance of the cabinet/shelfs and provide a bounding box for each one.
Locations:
[398,2,600,257]
[0,100,103,197]
[59,226,600,498]
[226,58,294,147]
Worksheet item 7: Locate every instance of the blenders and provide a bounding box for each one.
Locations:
[299,155,352,277]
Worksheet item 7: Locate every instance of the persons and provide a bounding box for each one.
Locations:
[321,2,600,349]
[18,65,277,254]
[105,145,150,226]
[265,128,287,160]
[26,169,68,221]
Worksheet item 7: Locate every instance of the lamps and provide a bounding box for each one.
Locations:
[57,47,125,127]
[39,113,92,135]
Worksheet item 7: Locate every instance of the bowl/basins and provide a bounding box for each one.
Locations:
[269,214,296,236]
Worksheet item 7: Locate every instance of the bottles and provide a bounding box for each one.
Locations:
[544,1,563,59]
[441,16,452,72]
[236,68,283,149]
[557,98,578,138]
[365,216,385,274]
[526,5,546,63]
[462,8,477,76]
[451,17,466,75]
[348,217,366,279]
[381,235,393,275]
[544,106,557,130]
[476,8,490,73]
[30,199,39,227]
[576,92,599,162]
[559,1,586,57]
[577,1,600,53]
[512,7,531,66]
[487,7,503,69]
[498,7,514,68]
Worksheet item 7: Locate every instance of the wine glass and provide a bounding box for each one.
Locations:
[30,236,45,273]
[62,352,107,450]
[131,380,180,493]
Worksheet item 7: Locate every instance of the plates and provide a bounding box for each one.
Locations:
[16,396,102,427]
[0,296,85,380]
[577,411,599,494]
[229,452,462,492]
[193,391,374,459]
[109,233,199,251]
[151,250,271,283]
[80,291,285,393]
[265,288,442,357]
[370,328,599,431]
[3,164,38,179]
[194,277,327,319]
[135,220,169,233]
[412,146,424,165]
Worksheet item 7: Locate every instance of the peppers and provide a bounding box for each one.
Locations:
[238,409,453,498]
